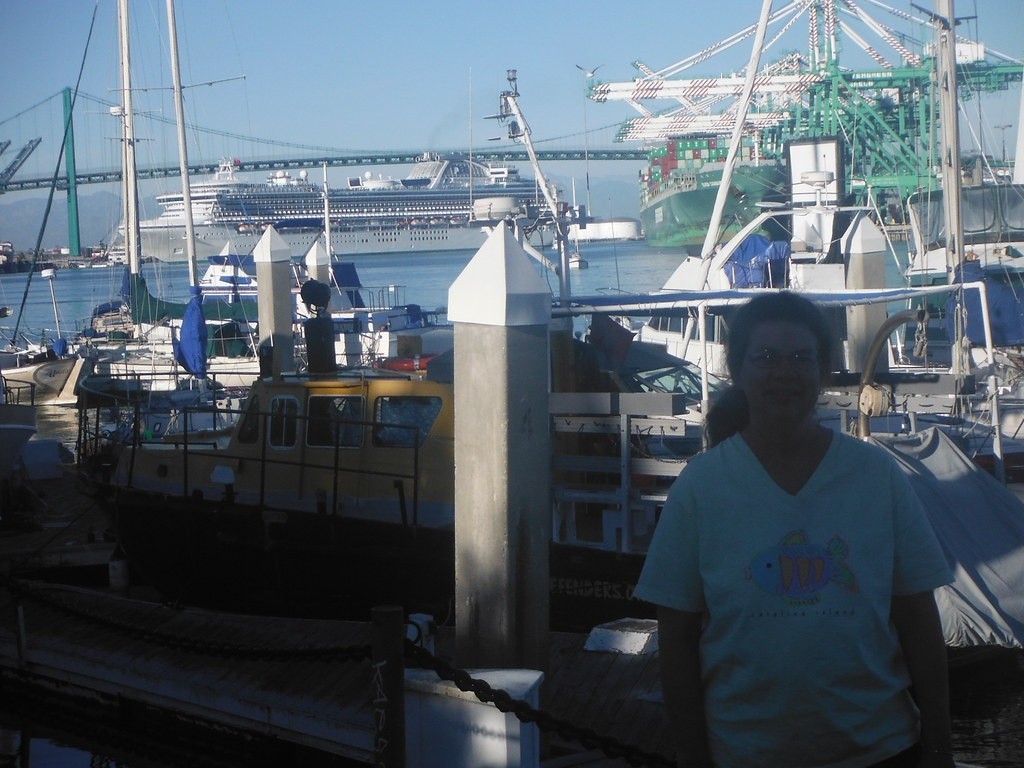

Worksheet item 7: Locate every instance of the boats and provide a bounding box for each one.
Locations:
[0,0,1024,558]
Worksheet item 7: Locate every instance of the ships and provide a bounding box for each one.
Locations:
[116,146,573,259]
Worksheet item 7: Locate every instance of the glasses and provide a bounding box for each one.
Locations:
[745,348,823,371]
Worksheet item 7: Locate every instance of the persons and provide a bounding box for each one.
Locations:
[703,383,752,448]
[627,291,959,768]
